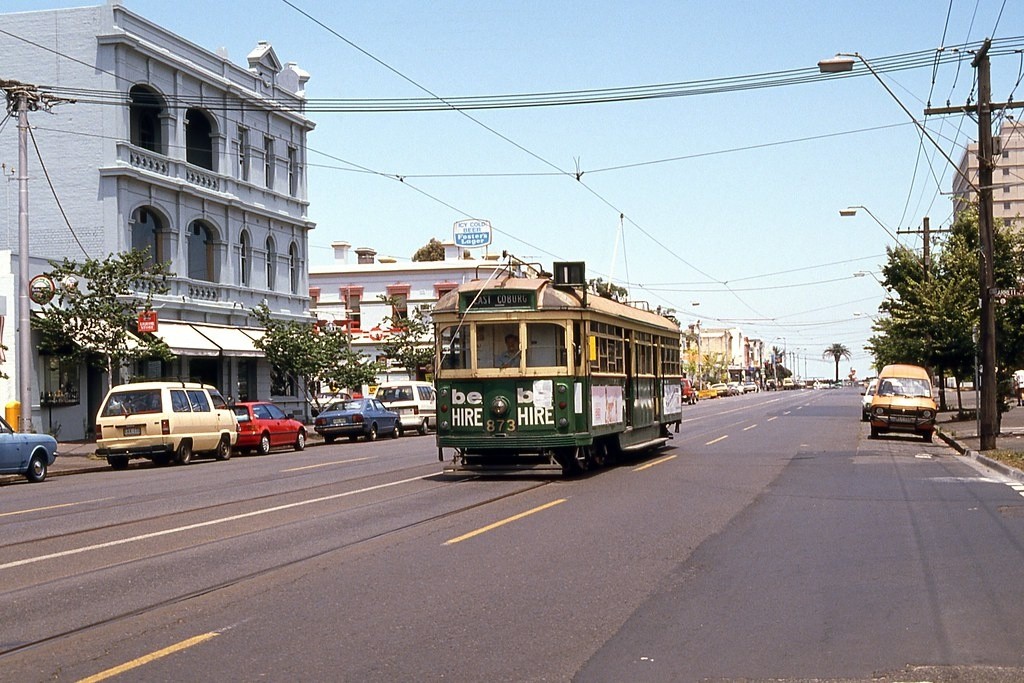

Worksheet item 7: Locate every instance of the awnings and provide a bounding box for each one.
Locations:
[34,312,153,358]
[130,317,322,361]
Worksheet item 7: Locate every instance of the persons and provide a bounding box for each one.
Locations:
[1013,367,1024,407]
[496,334,527,370]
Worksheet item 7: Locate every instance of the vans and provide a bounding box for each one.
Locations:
[373,380,437,435]
[95,382,239,470]
[869,365,938,442]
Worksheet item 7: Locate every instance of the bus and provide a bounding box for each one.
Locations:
[429,260,683,479]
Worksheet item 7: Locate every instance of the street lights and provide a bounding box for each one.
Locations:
[851,269,895,304]
[839,205,932,381]
[819,52,999,452]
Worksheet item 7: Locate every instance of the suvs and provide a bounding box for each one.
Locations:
[711,382,730,399]
[860,379,908,421]
[765,379,779,391]
[727,382,745,396]
[743,381,759,394]
[783,378,797,390]
[680,380,697,406]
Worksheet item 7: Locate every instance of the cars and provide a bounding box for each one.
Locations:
[0,416,60,483]
[794,380,807,388]
[215,401,308,454]
[314,398,401,442]
